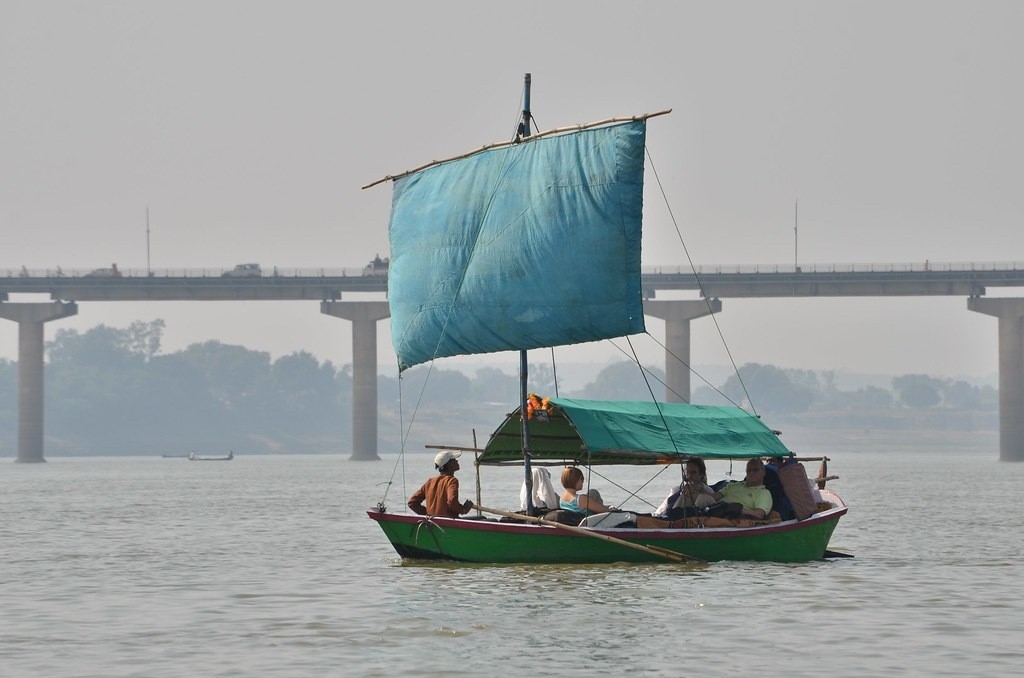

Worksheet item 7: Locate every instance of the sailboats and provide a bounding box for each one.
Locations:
[361,72,847,564]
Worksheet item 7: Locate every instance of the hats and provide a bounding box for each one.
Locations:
[435,449,462,469]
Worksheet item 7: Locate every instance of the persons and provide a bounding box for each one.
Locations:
[408,450,498,522]
[651,456,789,521]
[519,467,622,517]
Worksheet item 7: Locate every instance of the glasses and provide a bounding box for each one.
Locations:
[746,468,763,474]
